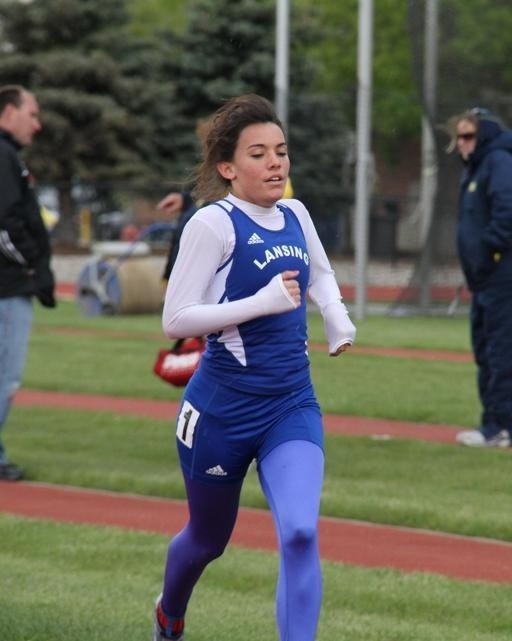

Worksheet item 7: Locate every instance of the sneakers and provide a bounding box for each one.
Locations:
[456,429,511,447]
[156,592,184,641]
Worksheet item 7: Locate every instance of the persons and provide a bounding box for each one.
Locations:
[154,93,356,640]
[447,109,512,450]
[0,84,55,481]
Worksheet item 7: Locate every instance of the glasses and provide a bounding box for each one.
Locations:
[457,134,475,139]
